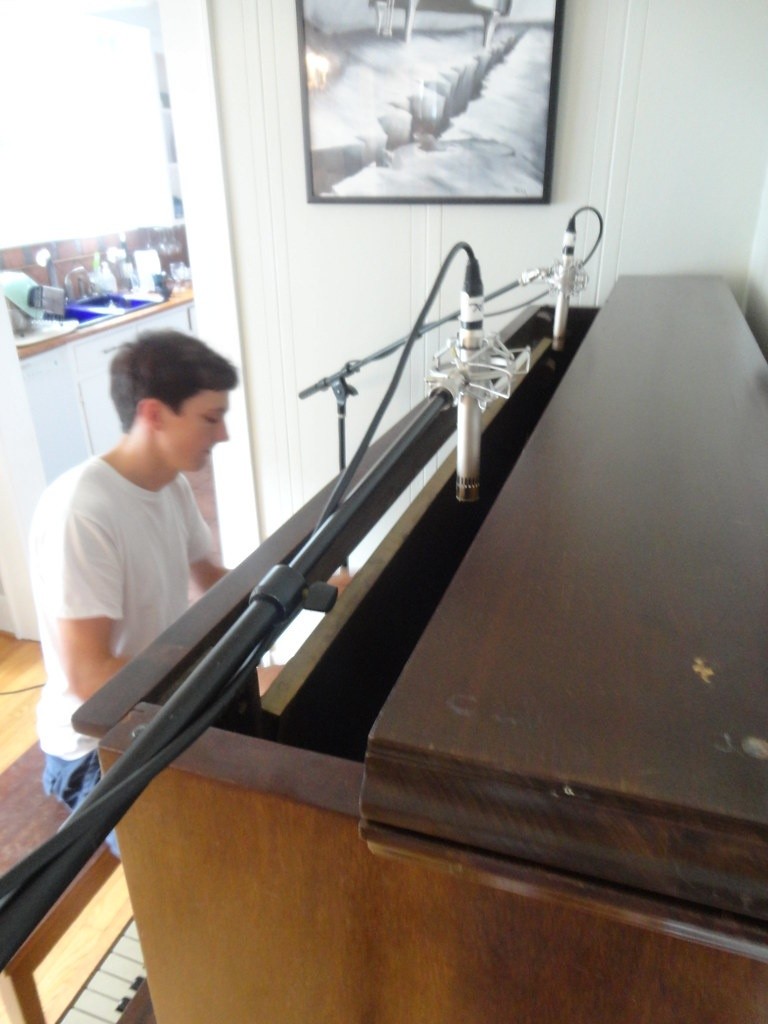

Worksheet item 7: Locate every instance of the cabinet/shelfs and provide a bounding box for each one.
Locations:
[18,293,197,491]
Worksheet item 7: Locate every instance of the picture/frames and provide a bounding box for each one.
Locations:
[294,0,569,206]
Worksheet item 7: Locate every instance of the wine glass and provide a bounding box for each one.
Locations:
[146,227,182,255]
[170,261,186,291]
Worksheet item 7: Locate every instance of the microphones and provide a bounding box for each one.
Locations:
[454,258,486,503]
[548,218,578,352]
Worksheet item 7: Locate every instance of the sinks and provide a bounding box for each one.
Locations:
[54,292,165,330]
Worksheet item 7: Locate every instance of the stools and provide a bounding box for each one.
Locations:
[0,739,128,1023]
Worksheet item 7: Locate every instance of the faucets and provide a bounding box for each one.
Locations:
[62,264,87,304]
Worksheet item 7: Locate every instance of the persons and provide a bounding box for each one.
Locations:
[29,330,356,856]
